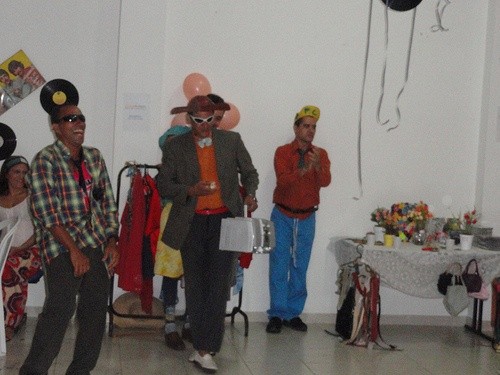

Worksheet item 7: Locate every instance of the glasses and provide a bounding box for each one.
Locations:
[190,115,214,124]
[57,114,86,122]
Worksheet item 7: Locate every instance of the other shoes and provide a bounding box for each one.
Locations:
[189,350,218,370]
[283,317,307,331]
[165,331,185,350]
[266,317,282,333]
[182,327,193,342]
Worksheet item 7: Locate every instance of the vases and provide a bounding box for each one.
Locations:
[375,226,386,241]
[383,234,393,245]
[459,233,474,250]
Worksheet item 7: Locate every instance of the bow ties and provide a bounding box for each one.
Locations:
[198,138,212,148]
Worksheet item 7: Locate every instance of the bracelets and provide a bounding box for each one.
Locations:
[248,193,257,202]
[107,235,119,244]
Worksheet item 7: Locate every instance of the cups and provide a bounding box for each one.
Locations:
[446,239,455,251]
[367,233,375,247]
[393,237,401,249]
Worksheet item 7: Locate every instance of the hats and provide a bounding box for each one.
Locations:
[207,93,231,111]
[170,96,217,113]
[294,106,321,122]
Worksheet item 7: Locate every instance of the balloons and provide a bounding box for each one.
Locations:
[171,73,240,130]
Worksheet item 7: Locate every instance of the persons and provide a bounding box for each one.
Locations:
[265,106,332,334]
[0,60,45,109]
[154,93,259,372]
[0,155,41,342]
[18,104,120,375]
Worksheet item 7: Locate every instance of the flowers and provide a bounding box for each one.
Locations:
[451,206,480,234]
[368,201,434,238]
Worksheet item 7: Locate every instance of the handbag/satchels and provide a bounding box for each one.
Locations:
[462,259,483,293]
[443,264,468,314]
[437,262,463,296]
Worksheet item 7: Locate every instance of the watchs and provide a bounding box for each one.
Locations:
[247,193,258,202]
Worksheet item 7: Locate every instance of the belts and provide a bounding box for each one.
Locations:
[275,202,318,214]
[196,208,229,215]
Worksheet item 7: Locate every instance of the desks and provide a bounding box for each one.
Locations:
[333,239,500,350]
